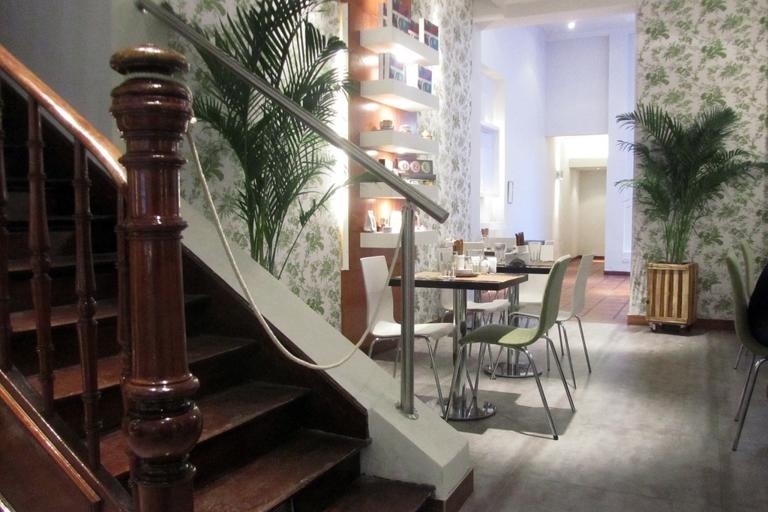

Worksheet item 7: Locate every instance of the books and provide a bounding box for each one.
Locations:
[378,0,440,94]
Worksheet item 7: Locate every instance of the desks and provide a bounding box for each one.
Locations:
[388,270,528,420]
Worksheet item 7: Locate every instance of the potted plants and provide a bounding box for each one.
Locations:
[609,104,755,331]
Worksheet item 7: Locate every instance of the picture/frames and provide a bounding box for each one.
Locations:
[508,182,513,204]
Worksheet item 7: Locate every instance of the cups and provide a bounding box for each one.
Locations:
[437,241,542,279]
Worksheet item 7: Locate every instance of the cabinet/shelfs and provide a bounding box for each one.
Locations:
[358,26,441,250]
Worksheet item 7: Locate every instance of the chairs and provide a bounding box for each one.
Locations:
[490,254,594,389]
[724,238,768,450]
[441,254,583,440]
[430,247,512,380]
[359,255,454,416]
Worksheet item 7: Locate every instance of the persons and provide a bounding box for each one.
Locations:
[746,263,768,349]
